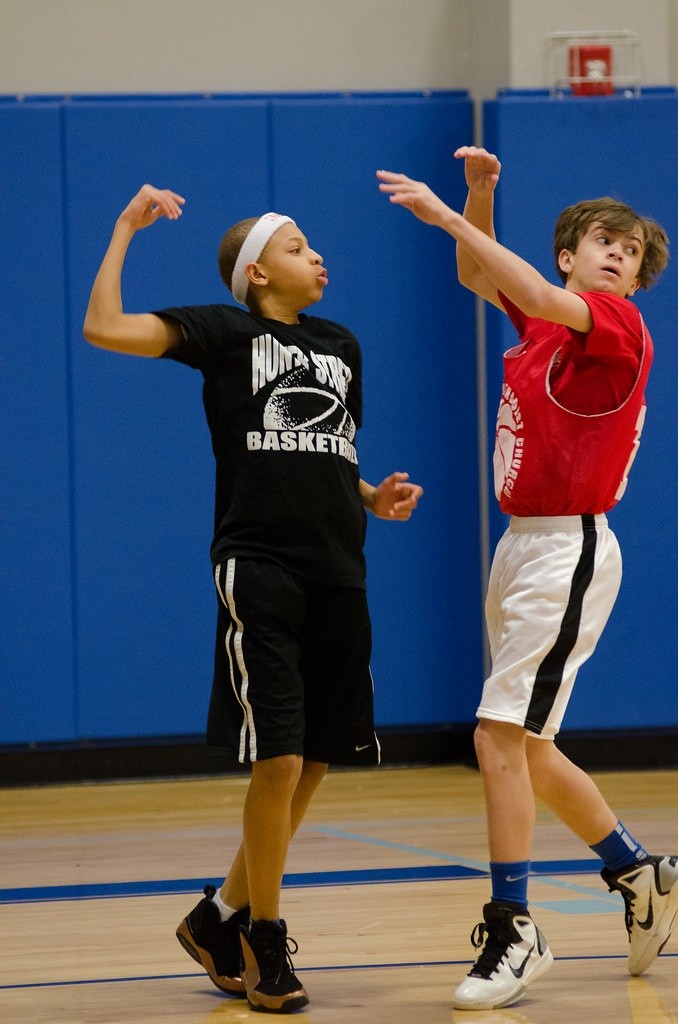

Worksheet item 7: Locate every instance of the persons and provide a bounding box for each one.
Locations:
[83,184,423,1013]
[376,146,678,1010]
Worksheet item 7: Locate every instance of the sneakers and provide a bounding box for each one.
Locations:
[239,919,310,1014]
[176,884,250,998]
[453,901,554,1011]
[600,855,678,976]
